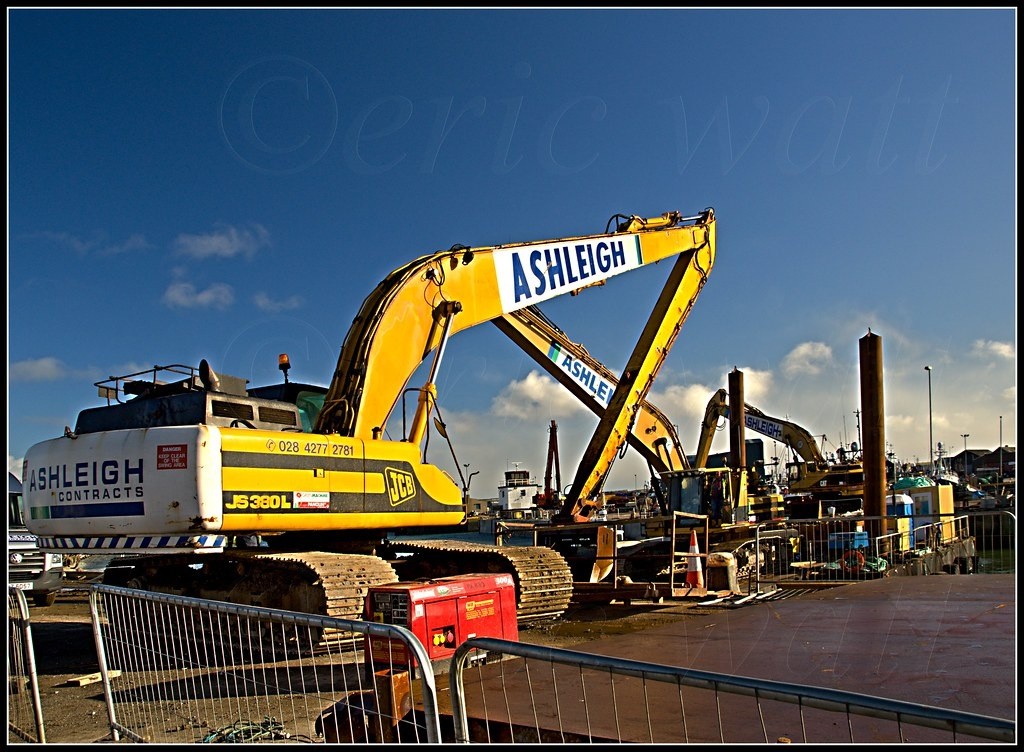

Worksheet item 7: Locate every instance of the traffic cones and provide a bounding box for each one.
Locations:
[685,530,706,589]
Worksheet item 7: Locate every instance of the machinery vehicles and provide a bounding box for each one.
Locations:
[20,207,719,667]
[488,304,961,581]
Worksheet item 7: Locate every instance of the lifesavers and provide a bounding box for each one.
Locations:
[611,506,615,511]
[841,550,865,572]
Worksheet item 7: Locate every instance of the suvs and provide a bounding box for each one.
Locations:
[9,471,63,605]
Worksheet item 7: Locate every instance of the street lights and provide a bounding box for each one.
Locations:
[999,415,1004,478]
[960,433,969,477]
[924,365,937,479]
